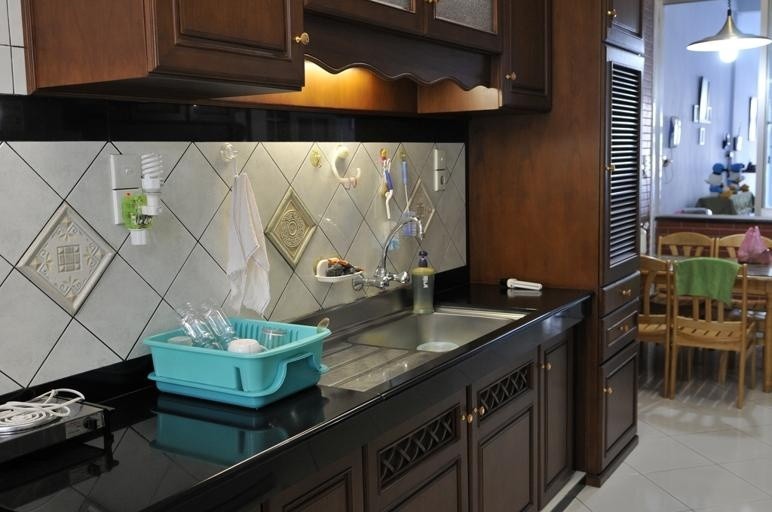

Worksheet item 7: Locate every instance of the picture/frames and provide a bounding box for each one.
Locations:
[669,116,682,149]
[698,127,706,145]
[699,75,711,124]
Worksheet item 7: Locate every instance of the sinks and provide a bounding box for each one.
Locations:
[340,303,530,353]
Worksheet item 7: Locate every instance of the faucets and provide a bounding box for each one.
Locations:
[351,216,426,292]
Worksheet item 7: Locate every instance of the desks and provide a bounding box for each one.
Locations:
[641,254,772,393]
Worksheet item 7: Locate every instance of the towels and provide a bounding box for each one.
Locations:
[226,172,271,318]
[673,257,743,308]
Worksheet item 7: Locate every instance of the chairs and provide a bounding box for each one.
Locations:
[712,232,772,331]
[651,231,715,316]
[639,255,684,398]
[669,257,758,409]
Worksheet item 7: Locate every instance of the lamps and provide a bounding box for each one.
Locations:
[686,0,772,64]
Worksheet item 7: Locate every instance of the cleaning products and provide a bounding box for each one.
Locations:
[411,251,434,314]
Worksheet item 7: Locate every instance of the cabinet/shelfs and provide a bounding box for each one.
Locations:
[539,326,577,508]
[20,1,310,94]
[466,0,642,488]
[500,0,553,113]
[242,444,363,512]
[361,347,540,512]
[303,0,501,88]
